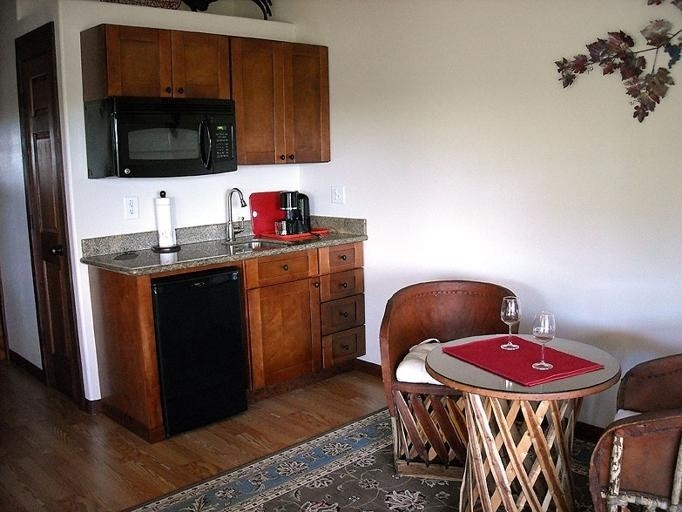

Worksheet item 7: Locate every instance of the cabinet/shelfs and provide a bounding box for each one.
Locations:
[230,35,331,166]
[79,22,231,102]
[243,248,322,398]
[318,240,366,379]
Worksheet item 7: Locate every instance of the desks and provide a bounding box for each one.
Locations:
[426,333,621,511]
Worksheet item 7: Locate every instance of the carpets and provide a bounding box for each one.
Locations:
[119,396,602,511]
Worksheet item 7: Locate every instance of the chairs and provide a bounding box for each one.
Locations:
[587,353,681,511]
[379,279,520,484]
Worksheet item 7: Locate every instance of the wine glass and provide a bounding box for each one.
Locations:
[530,310,556,371]
[500,296,522,350]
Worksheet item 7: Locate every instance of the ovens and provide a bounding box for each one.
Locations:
[150,265,248,435]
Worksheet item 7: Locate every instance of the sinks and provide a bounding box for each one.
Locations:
[245,241,287,251]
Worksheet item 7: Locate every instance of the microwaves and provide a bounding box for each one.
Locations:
[84,97,237,180]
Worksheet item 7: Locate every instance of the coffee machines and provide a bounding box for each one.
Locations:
[275,192,310,234]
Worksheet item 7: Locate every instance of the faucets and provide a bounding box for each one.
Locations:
[227,187,247,243]
